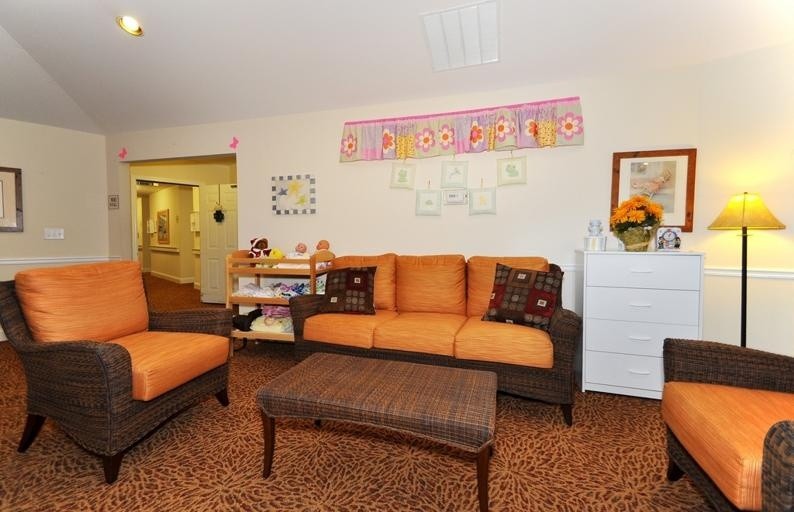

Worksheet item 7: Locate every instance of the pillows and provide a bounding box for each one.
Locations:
[467,256,549,318]
[481,262,564,332]
[396,255,467,315]
[319,266,377,315]
[331,253,396,309]
[15,261,149,343]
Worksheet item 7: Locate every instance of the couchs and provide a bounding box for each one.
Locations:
[289,264,583,426]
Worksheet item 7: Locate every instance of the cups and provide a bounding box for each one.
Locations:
[582,236,607,251]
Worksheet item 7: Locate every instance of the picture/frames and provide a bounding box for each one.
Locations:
[609,148,697,232]
[157,209,169,244]
[497,156,528,186]
[389,162,416,190]
[441,160,469,188]
[0,167,24,232]
[469,187,496,216]
[416,189,441,216]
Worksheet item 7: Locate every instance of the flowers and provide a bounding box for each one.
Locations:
[609,193,664,234]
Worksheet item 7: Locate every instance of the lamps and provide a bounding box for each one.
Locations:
[706,192,786,348]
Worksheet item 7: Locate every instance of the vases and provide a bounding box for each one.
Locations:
[615,221,658,252]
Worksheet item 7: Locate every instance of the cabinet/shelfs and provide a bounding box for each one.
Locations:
[226,250,335,357]
[582,251,704,400]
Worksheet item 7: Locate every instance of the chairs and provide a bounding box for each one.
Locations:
[0,280,234,484]
[661,338,794,512]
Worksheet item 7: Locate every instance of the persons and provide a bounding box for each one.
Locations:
[248,237,329,269]
